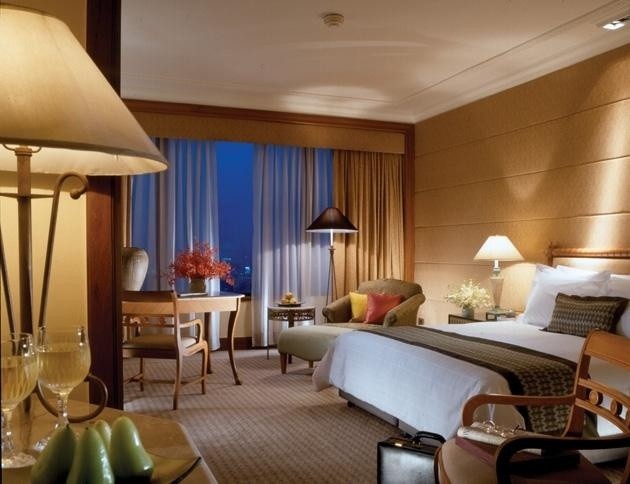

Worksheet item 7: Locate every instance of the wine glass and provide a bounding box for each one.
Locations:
[0,332,40,469]
[30,324,93,453]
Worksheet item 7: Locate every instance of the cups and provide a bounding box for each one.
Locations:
[478,417,516,438]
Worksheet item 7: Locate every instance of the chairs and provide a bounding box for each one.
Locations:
[434,330,630,484]
[320,279,426,330]
[122,289,209,411]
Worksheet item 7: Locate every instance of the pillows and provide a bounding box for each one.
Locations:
[524,263,630,337]
[348,291,368,322]
[364,292,402,325]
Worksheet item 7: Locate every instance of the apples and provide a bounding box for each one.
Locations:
[281,292,297,305]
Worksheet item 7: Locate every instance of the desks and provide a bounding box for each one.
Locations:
[123,292,246,385]
[0,396,217,483]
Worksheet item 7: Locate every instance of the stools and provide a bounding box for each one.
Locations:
[278,325,356,374]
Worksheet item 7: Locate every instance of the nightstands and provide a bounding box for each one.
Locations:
[448,309,523,324]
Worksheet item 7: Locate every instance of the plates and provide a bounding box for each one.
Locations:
[276,302,302,307]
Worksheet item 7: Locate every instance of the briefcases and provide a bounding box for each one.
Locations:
[377,431,446,483]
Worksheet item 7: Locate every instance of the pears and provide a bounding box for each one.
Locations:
[33,415,154,483]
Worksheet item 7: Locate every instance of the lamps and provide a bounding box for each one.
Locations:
[472,234,525,309]
[306,208,360,324]
[0,7,167,453]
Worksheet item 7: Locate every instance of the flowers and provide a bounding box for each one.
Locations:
[446,279,496,310]
[158,239,235,287]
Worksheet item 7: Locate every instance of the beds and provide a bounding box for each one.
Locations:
[312,242,630,464]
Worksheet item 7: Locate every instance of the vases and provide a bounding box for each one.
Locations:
[120,246,149,292]
[462,305,474,318]
[189,276,206,292]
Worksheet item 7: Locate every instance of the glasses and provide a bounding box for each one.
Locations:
[483,420,526,438]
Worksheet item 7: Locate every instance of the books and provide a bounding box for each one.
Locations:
[456,421,542,454]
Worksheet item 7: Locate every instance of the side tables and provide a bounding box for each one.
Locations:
[267,305,315,364]
